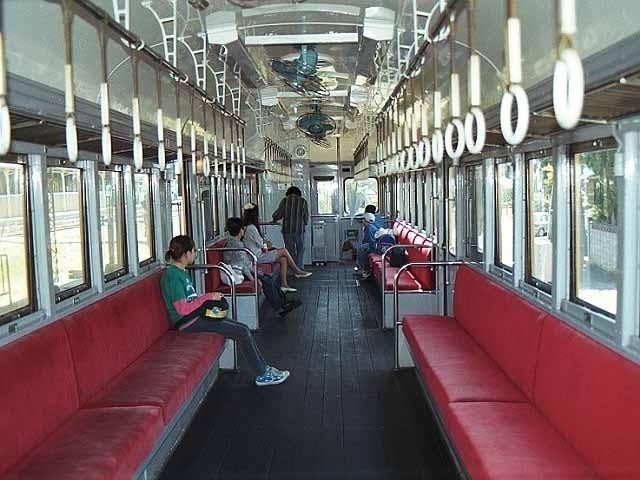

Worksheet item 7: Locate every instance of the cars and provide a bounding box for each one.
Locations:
[171,191,176,201]
[533,212,551,237]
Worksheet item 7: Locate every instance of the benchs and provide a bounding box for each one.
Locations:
[204,237,279,331]
[393,259,640,480]
[368,220,447,330]
[0,263,237,480]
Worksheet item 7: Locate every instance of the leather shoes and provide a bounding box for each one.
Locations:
[280,300,303,318]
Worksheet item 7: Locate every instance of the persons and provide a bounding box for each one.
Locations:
[271,186,308,271]
[357,213,378,280]
[223,218,302,320]
[160,236,290,386]
[364,205,385,231]
[245,204,313,293]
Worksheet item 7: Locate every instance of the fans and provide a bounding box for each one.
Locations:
[304,133,333,150]
[266,46,331,99]
[294,102,336,138]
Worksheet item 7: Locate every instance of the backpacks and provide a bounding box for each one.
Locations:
[375,234,396,255]
[200,297,229,320]
[218,262,244,286]
[390,247,408,267]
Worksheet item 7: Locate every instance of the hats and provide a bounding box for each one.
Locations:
[364,213,375,223]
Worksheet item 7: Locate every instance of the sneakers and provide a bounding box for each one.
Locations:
[360,271,373,280]
[256,366,290,386]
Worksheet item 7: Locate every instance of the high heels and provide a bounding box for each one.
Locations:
[281,287,297,293]
[296,272,312,279]
